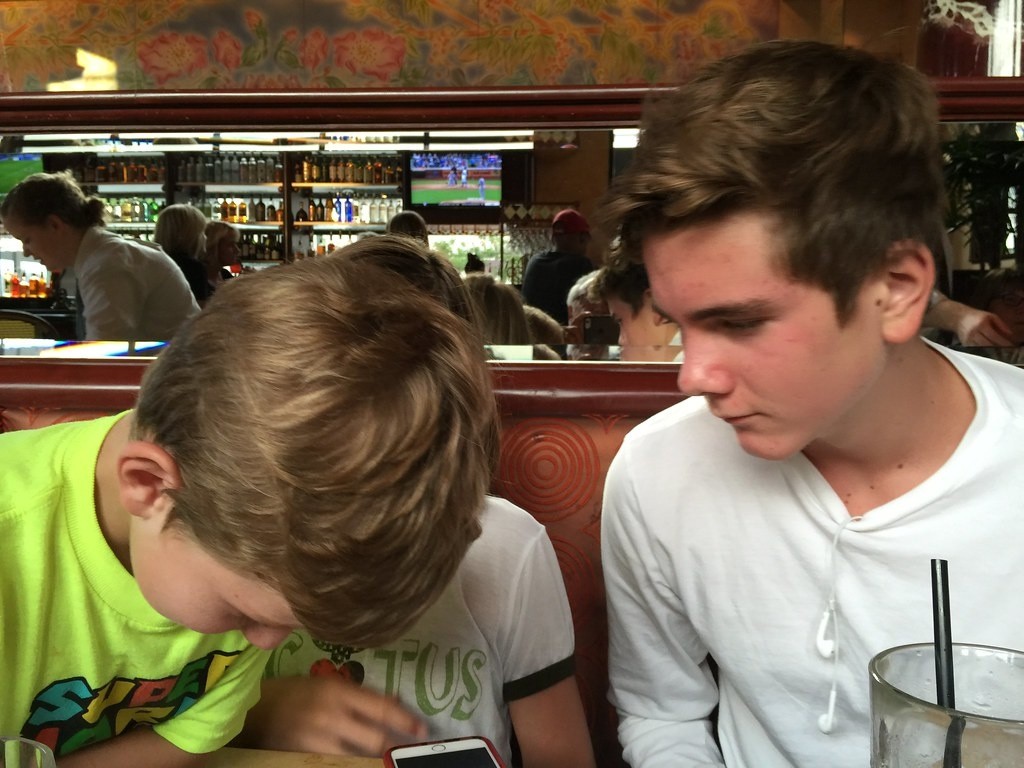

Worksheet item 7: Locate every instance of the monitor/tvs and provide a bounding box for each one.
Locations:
[0,152,52,208]
[403,150,508,225]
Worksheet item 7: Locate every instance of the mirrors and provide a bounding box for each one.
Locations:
[0,77,1024,417]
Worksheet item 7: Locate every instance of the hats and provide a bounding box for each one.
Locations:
[552,210,590,234]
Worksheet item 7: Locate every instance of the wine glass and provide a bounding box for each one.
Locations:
[504,229,552,254]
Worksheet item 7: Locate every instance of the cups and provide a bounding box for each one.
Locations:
[0,735,57,768]
[868,641,1024,768]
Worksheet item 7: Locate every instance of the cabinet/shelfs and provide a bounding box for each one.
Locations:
[43,153,406,264]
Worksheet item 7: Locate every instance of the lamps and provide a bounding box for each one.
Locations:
[91,184,402,230]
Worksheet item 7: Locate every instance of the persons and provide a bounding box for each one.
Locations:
[410,153,502,205]
[1,172,242,341]
[386,209,608,358]
[598,38,1024,768]
[0,233,591,768]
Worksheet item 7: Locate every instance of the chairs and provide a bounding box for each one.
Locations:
[1,309,59,341]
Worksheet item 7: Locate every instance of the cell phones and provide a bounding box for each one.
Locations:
[384,735,508,768]
[584,314,621,346]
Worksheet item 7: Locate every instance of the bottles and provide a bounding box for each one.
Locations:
[95,157,166,243]
[174,153,282,259]
[288,154,402,260]
[3,269,47,298]
